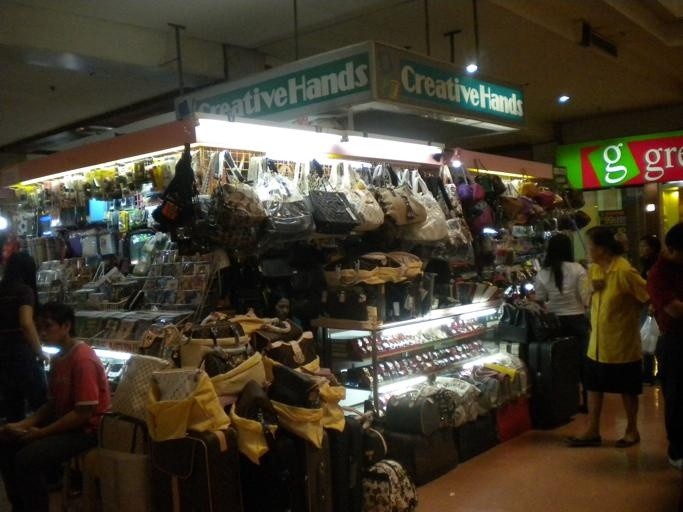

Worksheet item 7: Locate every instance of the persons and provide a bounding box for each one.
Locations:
[265,291,308,332]
[0,300,114,510]
[0,252,65,491]
[527,223,683,470]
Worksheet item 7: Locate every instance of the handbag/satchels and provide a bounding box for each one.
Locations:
[497,302,560,342]
[322,252,438,321]
[386,362,527,436]
[151,174,591,255]
[113,309,346,465]
[361,459,418,511]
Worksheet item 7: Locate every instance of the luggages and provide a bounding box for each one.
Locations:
[84,411,388,512]
[383,393,531,487]
[499,337,577,430]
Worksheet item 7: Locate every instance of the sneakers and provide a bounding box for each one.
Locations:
[617,436,641,445]
[563,435,602,446]
[669,458,683,468]
